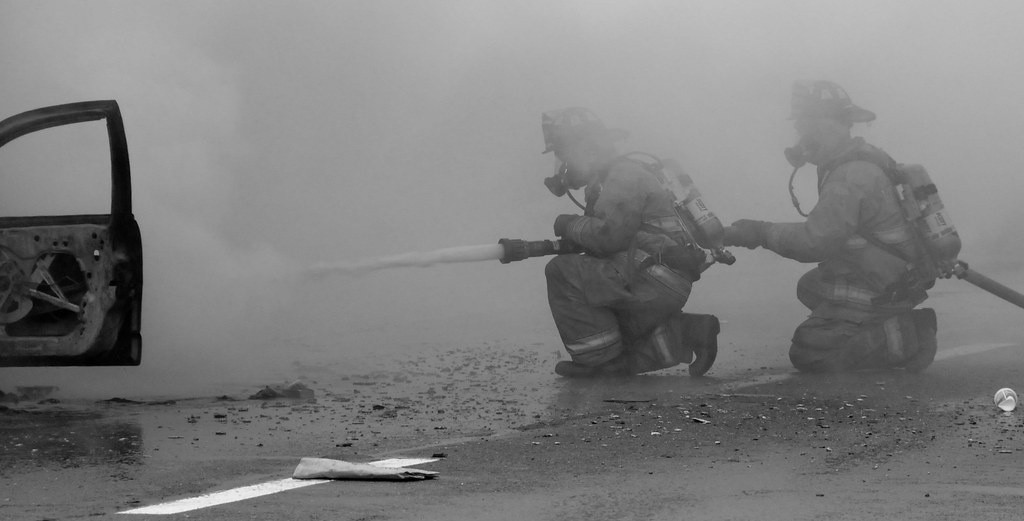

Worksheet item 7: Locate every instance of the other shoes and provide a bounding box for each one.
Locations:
[906,308,937,372]
[685,314,720,377]
[555,352,630,378]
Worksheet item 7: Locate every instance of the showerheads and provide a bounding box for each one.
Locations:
[496,237,559,263]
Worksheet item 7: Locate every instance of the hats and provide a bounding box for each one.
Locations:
[540,108,603,154]
[787,79,876,124]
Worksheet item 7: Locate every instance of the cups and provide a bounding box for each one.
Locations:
[993,387,1018,412]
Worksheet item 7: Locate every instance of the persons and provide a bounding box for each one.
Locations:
[542,107,719,378]
[730,79,938,375]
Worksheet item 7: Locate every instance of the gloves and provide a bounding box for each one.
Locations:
[723,220,773,250]
[554,215,579,235]
